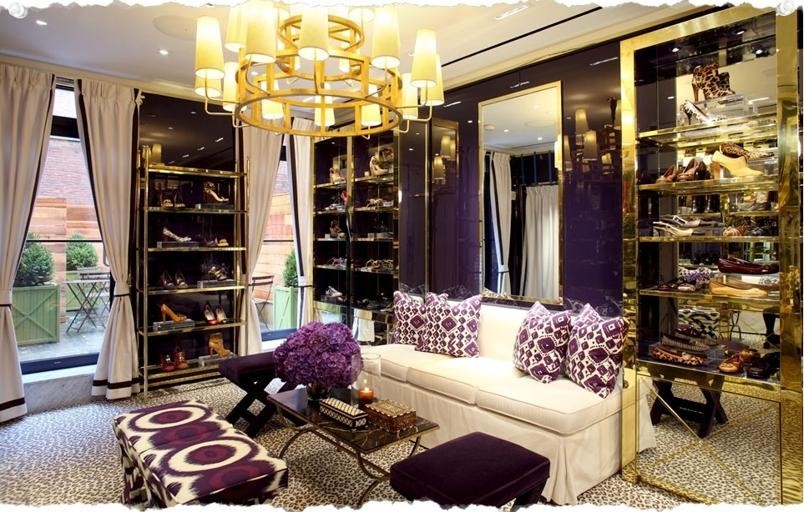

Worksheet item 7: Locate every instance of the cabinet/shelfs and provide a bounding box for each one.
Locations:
[130,91,252,388]
[618,0,799,382]
[308,128,399,314]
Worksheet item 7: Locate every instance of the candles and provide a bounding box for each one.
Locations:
[357,385,373,403]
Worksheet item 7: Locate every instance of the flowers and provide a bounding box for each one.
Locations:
[269,319,366,396]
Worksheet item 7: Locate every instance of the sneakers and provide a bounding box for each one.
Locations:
[324,284,394,312]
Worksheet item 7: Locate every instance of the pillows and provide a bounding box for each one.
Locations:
[412,292,483,357]
[555,302,632,399]
[388,291,449,345]
[511,297,570,383]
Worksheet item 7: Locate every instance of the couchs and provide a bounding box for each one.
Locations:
[637,374,658,455]
[347,288,619,505]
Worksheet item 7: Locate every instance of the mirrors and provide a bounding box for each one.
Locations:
[633,376,781,505]
[476,79,564,309]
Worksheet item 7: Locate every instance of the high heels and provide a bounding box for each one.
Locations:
[651,213,713,237]
[158,259,231,372]
[329,155,389,183]
[711,142,775,180]
[697,161,711,181]
[682,61,732,127]
[159,180,232,249]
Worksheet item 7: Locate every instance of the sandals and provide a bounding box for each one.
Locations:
[318,195,393,211]
[326,256,393,272]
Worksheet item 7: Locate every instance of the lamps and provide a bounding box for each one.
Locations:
[191,2,454,141]
[424,130,459,196]
[561,98,623,192]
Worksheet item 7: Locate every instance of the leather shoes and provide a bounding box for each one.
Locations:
[328,219,393,239]
[657,257,774,299]
[722,220,779,237]
[726,255,780,274]
[703,71,736,101]
[738,199,772,211]
[670,327,719,347]
[651,332,778,381]
[656,157,700,183]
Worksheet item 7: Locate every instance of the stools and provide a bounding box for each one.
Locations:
[139,427,290,507]
[107,396,233,505]
[648,366,728,442]
[389,428,552,511]
[216,350,297,440]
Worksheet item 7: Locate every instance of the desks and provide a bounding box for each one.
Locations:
[62,267,109,337]
